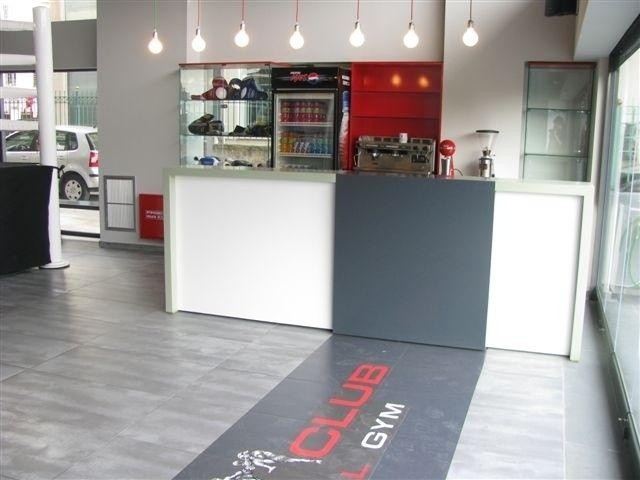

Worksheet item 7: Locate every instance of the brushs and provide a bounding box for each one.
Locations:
[352,136,436,178]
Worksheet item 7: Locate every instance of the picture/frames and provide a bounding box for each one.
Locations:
[162,167,600,363]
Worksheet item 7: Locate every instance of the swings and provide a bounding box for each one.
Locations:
[476,130,499,177]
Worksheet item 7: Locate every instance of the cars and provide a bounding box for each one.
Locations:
[399,133,407,144]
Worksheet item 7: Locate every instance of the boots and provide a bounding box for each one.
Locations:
[5,125,99,200]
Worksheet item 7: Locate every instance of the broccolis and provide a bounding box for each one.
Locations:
[271,66,351,172]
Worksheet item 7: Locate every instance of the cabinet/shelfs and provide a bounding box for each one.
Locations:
[282,128,329,153]
[282,101,327,122]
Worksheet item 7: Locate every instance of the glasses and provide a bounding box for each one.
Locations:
[290,0,305,48]
[235,0,249,48]
[403,0,419,48]
[149,0,163,55]
[192,0,206,52]
[349,0,364,47]
[462,0,478,46]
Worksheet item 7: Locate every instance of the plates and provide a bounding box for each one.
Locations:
[181,60,273,170]
[520,61,594,181]
[349,61,443,173]
